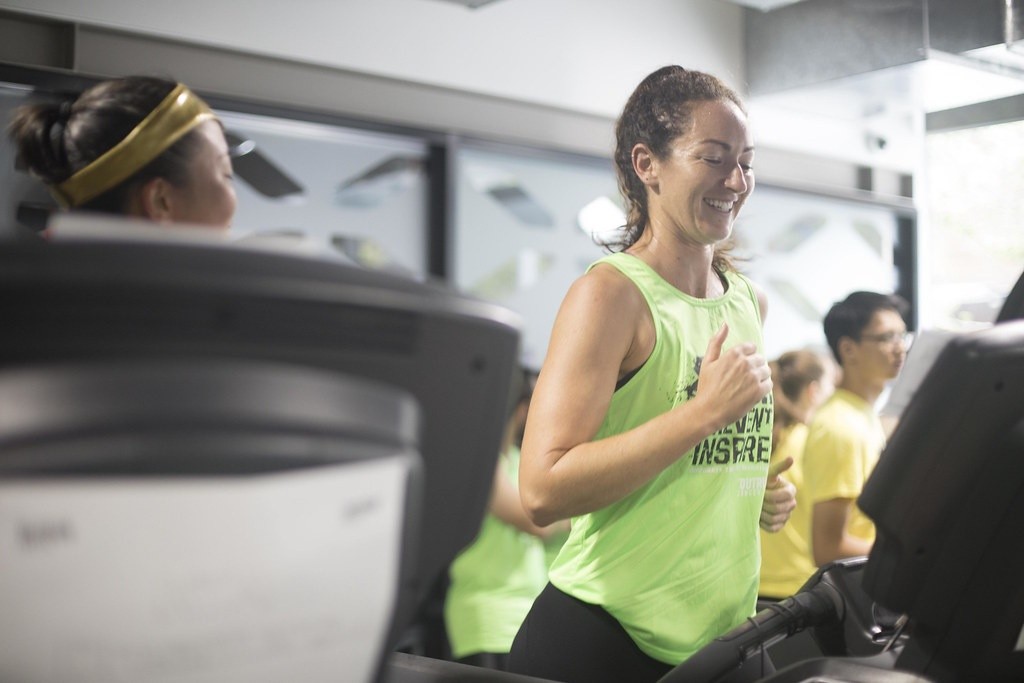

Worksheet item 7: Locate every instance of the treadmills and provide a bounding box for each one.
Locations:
[2,202,1024,683]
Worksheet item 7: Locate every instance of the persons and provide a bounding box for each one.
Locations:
[445,366,575,673]
[1,74,306,244]
[757,292,905,597]
[503,63,798,683]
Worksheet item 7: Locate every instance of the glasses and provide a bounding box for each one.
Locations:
[861,332,914,351]
